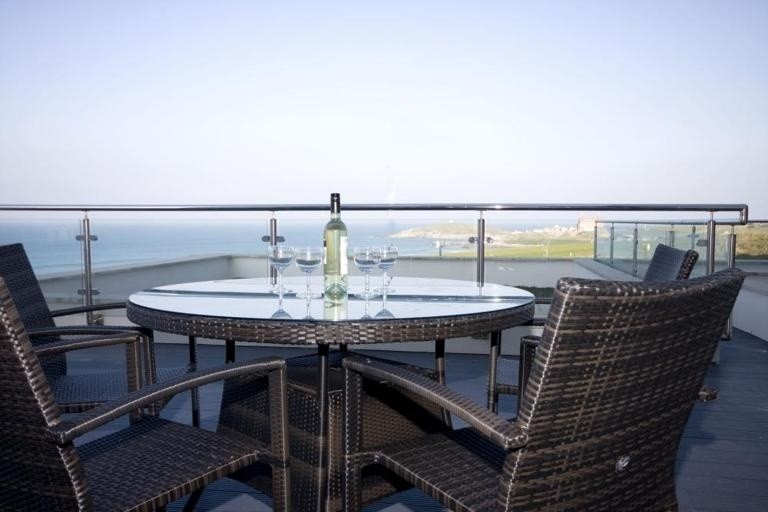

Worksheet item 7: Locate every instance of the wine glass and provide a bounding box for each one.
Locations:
[296,297,317,320]
[354,247,380,298]
[266,245,294,295]
[359,294,372,320]
[271,295,292,318]
[377,247,399,292]
[294,250,324,298]
[375,294,394,318]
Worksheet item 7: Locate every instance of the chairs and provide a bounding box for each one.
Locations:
[0,242,201,427]
[0,276,293,512]
[485,244,699,422]
[341,266,747,512]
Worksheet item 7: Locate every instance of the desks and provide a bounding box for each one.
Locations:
[126,275,535,512]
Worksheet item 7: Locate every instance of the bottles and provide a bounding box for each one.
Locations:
[323,293,348,321]
[322,193,349,293]
